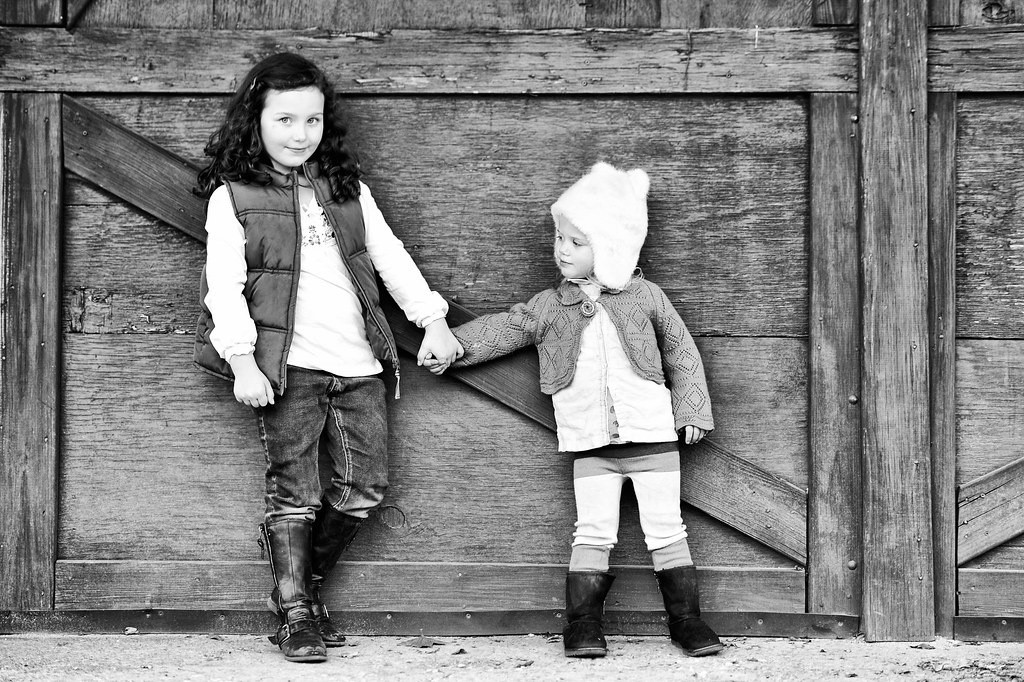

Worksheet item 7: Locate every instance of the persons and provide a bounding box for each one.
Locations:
[193,52,463,666]
[420,165,722,657]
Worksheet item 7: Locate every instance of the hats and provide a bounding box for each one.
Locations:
[550,159,650,292]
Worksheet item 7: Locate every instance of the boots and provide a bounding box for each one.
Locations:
[265,495,366,647]
[653,565,723,657]
[563,565,616,657]
[257,515,327,663]
